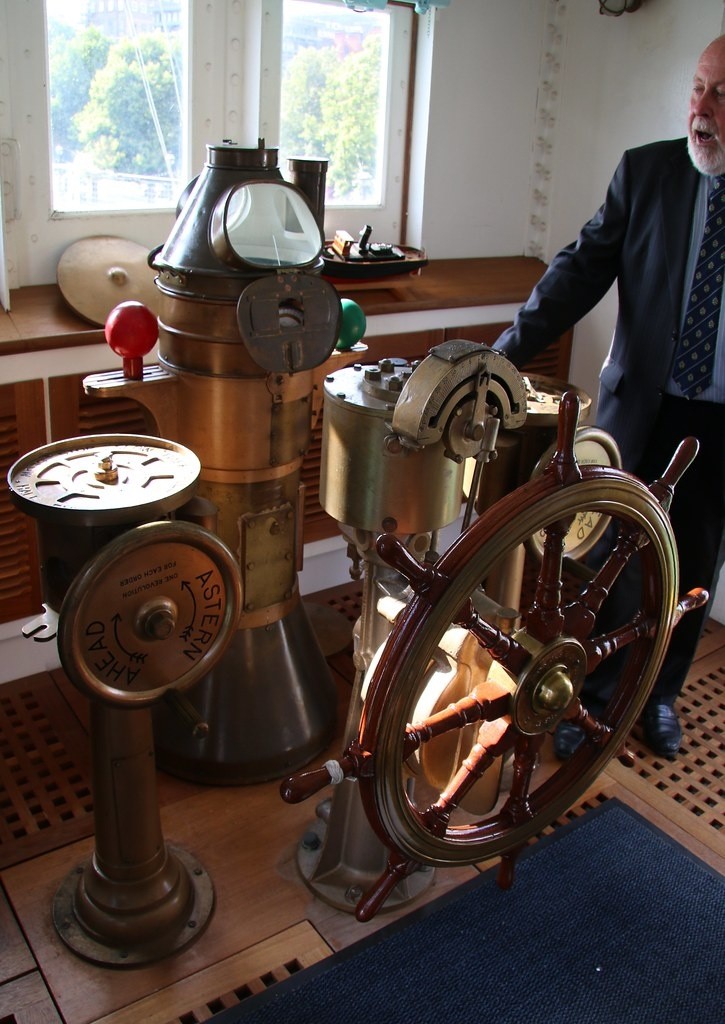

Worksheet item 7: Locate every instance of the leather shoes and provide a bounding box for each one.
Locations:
[554,716,584,762]
[643,703,682,761]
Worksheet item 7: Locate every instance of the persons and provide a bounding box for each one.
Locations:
[490,34,725,766]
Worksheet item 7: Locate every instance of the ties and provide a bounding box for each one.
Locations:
[672,173,725,400]
